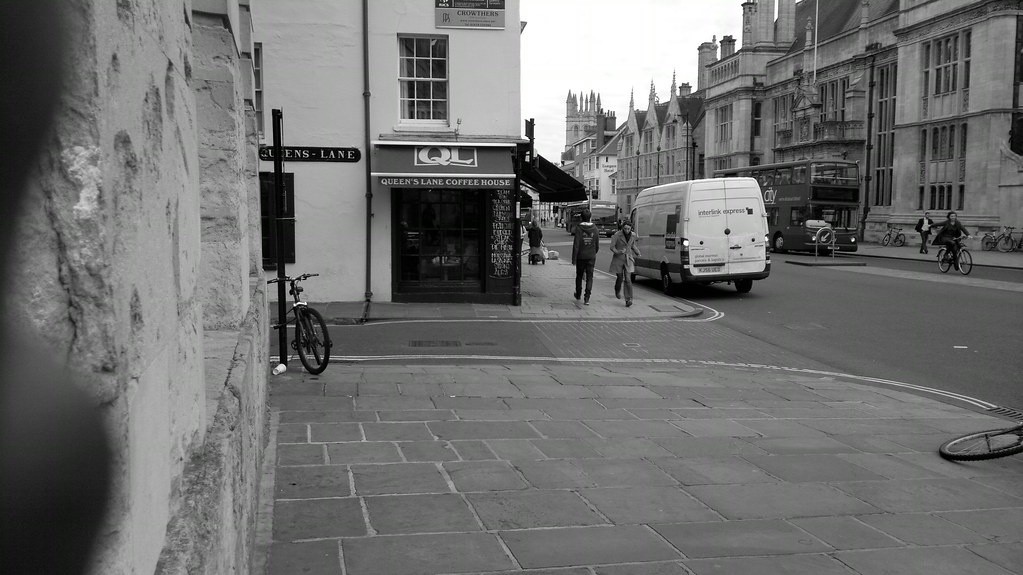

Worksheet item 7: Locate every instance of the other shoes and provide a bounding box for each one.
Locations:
[956,264,962,271]
[625,300,632,308]
[583,296,589,304]
[919,251,924,254]
[574,292,581,300]
[926,250,928,254]
[943,252,948,259]
[615,289,621,299]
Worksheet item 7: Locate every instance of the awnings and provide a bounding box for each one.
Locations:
[520,190,533,213]
[518,149,587,203]
[370,139,518,190]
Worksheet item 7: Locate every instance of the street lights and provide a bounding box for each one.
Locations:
[672,112,689,181]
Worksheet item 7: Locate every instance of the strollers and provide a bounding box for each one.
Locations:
[528,239,545,265]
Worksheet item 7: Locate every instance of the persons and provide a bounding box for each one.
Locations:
[914,212,940,254]
[520,220,526,251]
[527,221,543,265]
[572,209,600,305]
[927,211,971,272]
[608,221,641,307]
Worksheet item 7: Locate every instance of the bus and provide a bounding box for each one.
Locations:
[565,200,622,239]
[713,158,860,257]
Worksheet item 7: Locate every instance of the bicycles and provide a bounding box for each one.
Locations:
[882,225,905,247]
[267,273,333,374]
[936,236,974,275]
[981,226,1013,253]
[1001,226,1023,253]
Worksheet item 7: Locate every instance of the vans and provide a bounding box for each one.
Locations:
[629,177,771,299]
